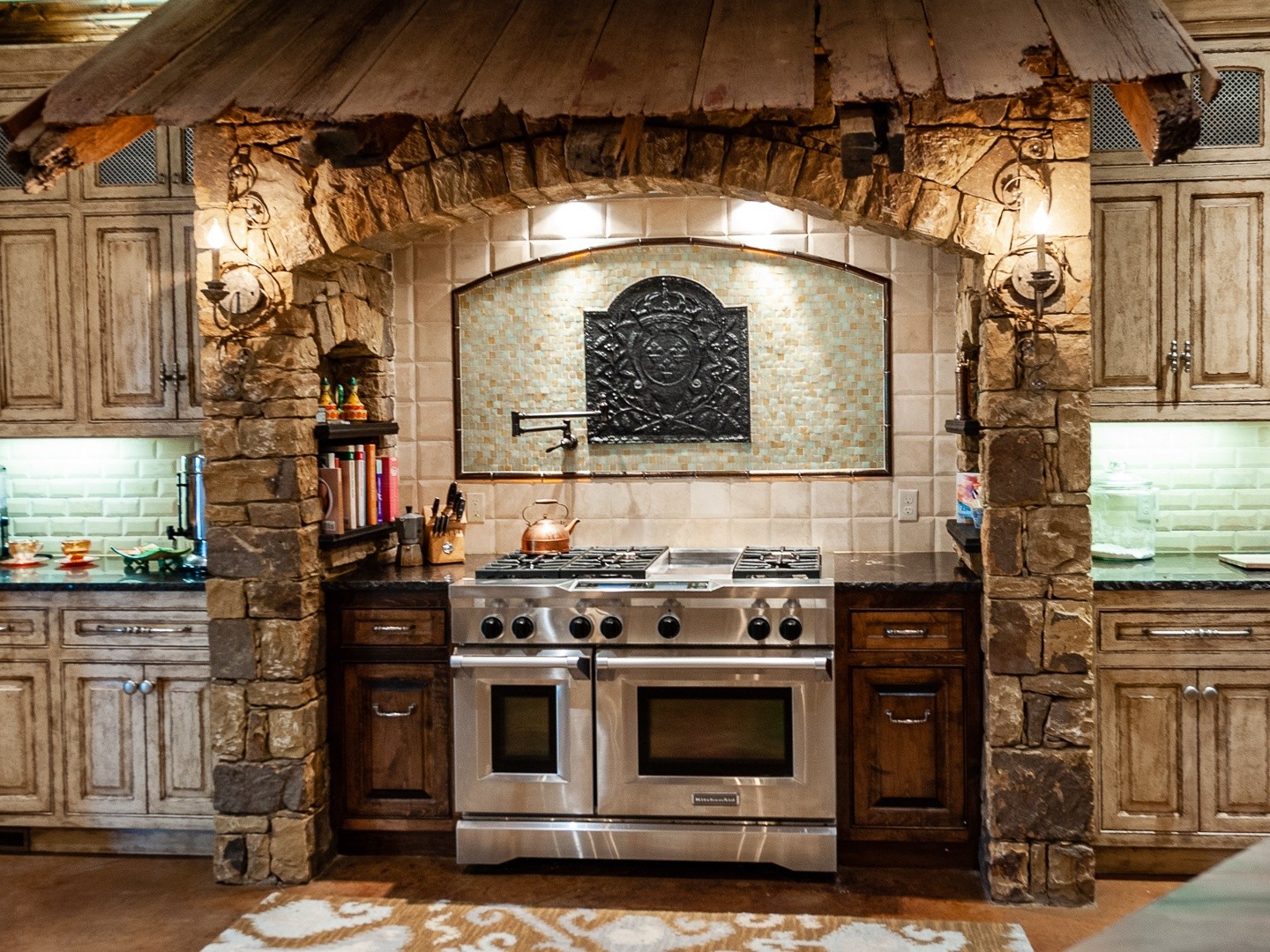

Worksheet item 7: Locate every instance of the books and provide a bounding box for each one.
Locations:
[175,446,207,576]
[954,471,980,524]
[1218,553,1270,568]
[315,439,405,537]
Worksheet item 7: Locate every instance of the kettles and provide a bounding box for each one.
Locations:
[520,499,581,556]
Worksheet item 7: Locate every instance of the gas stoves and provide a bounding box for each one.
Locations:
[449,543,836,651]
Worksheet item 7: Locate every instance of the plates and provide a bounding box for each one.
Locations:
[107,544,193,562]
[1,556,48,565]
[55,558,100,565]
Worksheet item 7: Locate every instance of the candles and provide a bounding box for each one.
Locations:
[1030,205,1050,269]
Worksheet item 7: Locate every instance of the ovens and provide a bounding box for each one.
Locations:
[452,643,837,827]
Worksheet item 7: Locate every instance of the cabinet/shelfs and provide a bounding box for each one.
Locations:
[823,542,980,880]
[2,548,218,864]
[1085,15,1270,425]
[315,420,401,544]
[0,39,208,443]
[1091,552,1270,882]
[333,545,508,861]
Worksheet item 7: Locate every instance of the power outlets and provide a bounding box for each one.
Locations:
[897,489,919,525]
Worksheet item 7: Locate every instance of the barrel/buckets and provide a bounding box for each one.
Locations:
[173,450,206,560]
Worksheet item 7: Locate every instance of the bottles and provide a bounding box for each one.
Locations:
[1088,461,1155,559]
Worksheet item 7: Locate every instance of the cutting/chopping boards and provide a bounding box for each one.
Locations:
[1217,552,1270,570]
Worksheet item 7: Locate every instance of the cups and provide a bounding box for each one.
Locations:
[7,538,42,560]
[60,539,92,561]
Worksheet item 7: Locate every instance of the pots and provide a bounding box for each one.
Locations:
[395,506,423,545]
